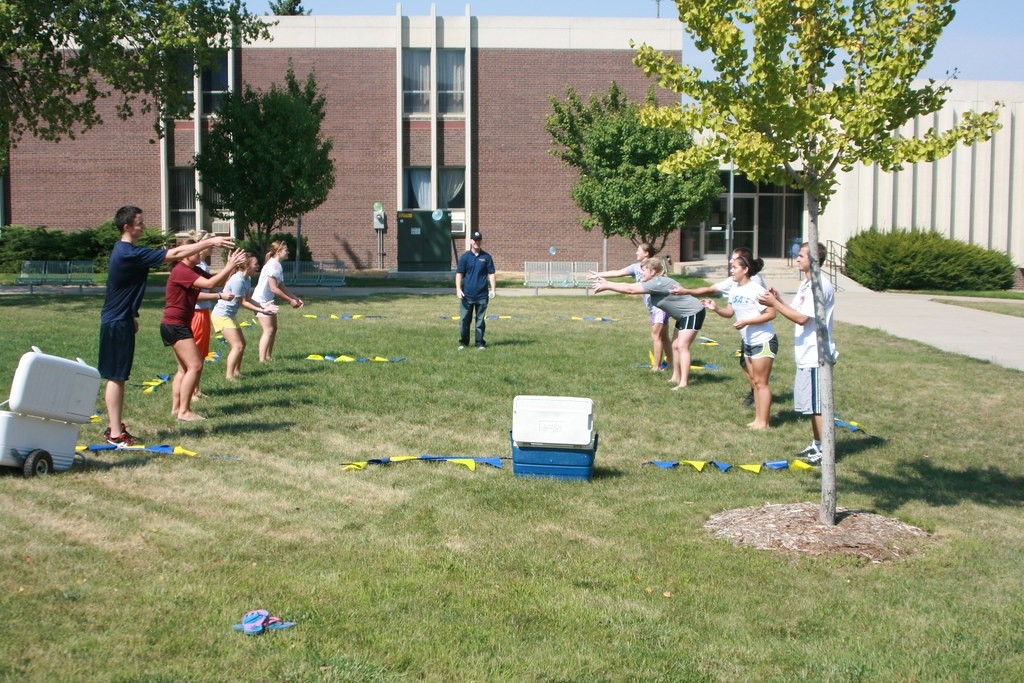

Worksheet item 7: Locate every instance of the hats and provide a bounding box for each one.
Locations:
[471,230,482,241]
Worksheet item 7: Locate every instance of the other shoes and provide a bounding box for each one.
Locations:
[744,391,754,406]
[479,346,485,349]
[458,345,464,350]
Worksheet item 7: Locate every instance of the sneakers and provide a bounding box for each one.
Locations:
[103,423,126,437]
[793,439,819,459]
[106,431,143,448]
[808,450,823,465]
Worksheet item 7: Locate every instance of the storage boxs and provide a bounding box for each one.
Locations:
[0,345,102,473]
[510,395,599,482]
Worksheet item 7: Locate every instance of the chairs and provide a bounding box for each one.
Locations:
[280,260,347,299]
[15,260,93,294]
[523,261,598,296]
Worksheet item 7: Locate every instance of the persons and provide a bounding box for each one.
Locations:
[593,258,706,391]
[755,242,838,467]
[700,251,778,430]
[211,252,279,381]
[97,206,235,446]
[188,230,235,401]
[252,240,304,363]
[456,231,496,350]
[160,240,246,421]
[586,242,672,371]
[670,247,767,406]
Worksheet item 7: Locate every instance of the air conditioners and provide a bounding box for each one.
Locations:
[211,222,230,235]
[450,221,466,236]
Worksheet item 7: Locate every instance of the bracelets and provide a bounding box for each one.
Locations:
[219,293,221,299]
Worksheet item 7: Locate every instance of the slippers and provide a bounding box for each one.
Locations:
[233,616,297,630]
[241,609,269,634]
